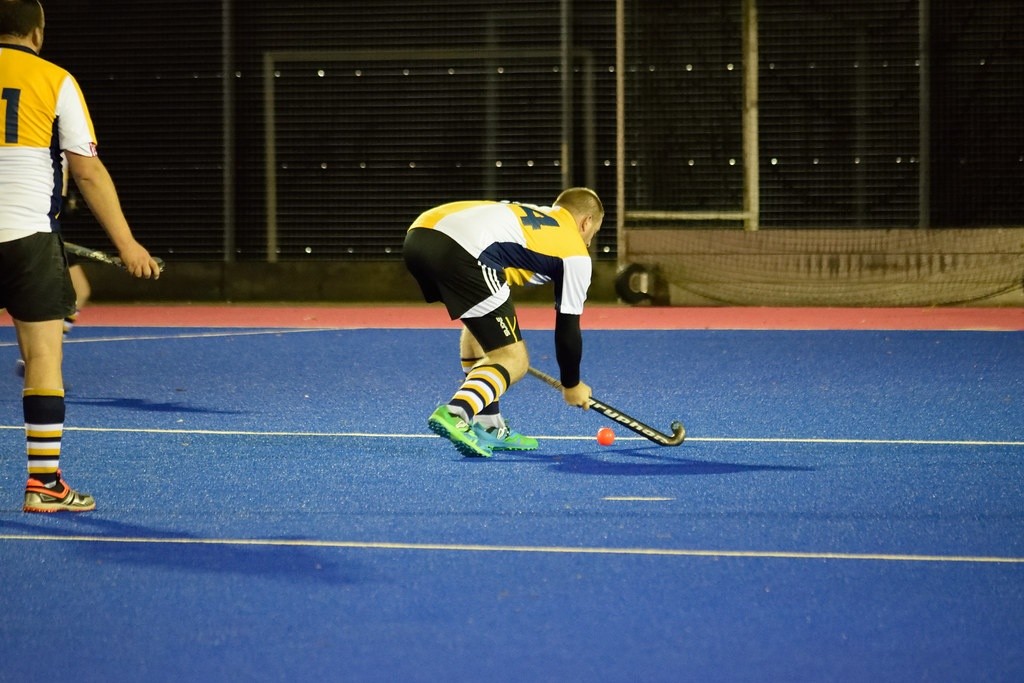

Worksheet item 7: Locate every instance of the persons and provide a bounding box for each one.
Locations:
[0,0,160,512]
[404,187,604,456]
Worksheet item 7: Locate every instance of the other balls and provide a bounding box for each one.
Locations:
[596,427,615,447]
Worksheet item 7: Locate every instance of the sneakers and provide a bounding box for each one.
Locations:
[23,469,96,513]
[426,405,493,458]
[473,421,538,451]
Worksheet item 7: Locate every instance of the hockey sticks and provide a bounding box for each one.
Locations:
[63,240,167,278]
[529,368,687,447]
